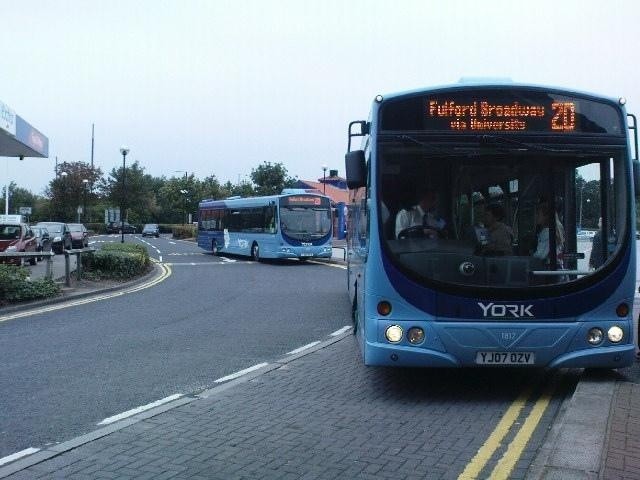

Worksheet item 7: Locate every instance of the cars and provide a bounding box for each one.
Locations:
[142,224,160,238]
[106,221,137,234]
[0,221,88,267]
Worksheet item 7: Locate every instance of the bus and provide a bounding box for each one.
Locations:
[197,188,338,263]
[344,80,640,369]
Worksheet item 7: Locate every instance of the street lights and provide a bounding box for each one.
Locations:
[321,164,329,195]
[82,179,89,223]
[180,190,188,226]
[120,144,131,243]
[60,172,69,222]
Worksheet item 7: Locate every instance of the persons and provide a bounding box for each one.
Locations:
[529,206,566,283]
[394,188,448,240]
[588,215,616,273]
[480,202,514,257]
